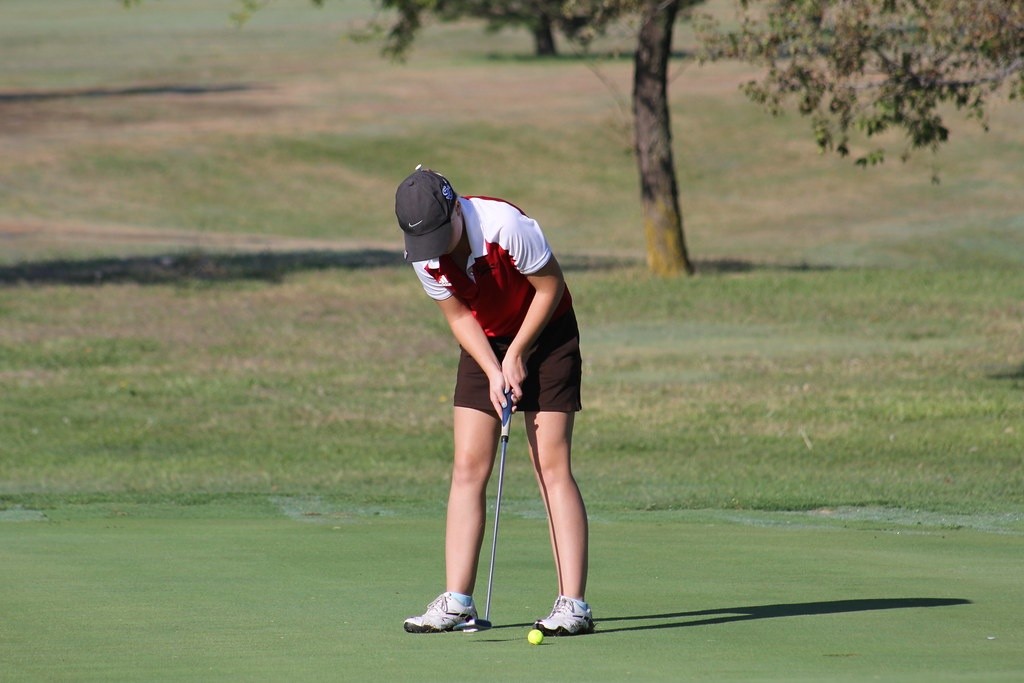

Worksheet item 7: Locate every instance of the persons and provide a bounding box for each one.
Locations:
[394,164,596,636]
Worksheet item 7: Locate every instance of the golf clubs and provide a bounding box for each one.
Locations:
[452,386,515,629]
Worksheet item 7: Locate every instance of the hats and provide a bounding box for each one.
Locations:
[395,169,457,262]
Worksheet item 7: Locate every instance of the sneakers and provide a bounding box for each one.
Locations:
[404,592,478,632]
[534,596,595,636]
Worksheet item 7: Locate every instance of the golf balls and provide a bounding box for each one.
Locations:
[527,629,543,645]
